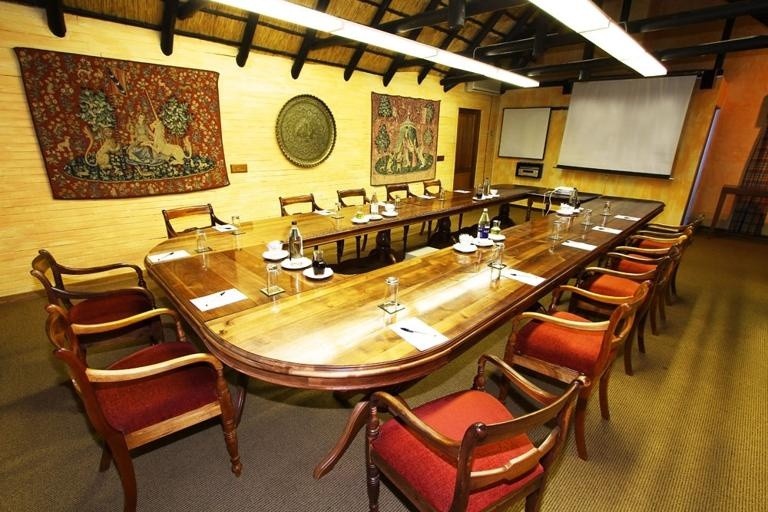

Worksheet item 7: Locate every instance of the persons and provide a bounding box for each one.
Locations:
[129,111,153,161]
[386,128,425,173]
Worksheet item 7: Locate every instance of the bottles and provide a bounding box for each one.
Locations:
[477,208,501,243]
[371,191,378,217]
[289,221,303,263]
[475,177,491,198]
[570,187,580,209]
[311,250,326,275]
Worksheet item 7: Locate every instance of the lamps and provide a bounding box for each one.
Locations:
[209,0,541,88]
[525,0,668,78]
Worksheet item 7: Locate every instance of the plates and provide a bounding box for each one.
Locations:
[303,267,333,280]
[472,194,500,201]
[556,208,584,215]
[282,258,312,270]
[351,211,397,223]
[454,233,506,254]
[262,250,289,261]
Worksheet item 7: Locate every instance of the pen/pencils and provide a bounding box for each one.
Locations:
[158,252,174,261]
[225,228,231,229]
[206,291,226,306]
[401,327,436,337]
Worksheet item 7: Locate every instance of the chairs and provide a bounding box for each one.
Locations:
[29,249,186,367]
[364,352,588,511]
[603,214,703,333]
[422,179,464,233]
[162,204,230,238]
[571,250,671,377]
[495,280,652,462]
[385,183,428,246]
[279,192,340,266]
[337,188,378,260]
[42,304,247,511]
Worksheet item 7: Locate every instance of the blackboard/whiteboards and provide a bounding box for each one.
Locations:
[498,107,551,160]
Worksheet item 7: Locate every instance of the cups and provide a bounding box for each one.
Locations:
[384,205,395,213]
[267,242,282,255]
[492,242,505,268]
[334,202,342,217]
[489,189,497,195]
[197,231,208,251]
[583,201,612,226]
[440,189,446,200]
[232,216,241,234]
[265,262,280,294]
[395,195,400,208]
[552,220,564,239]
[383,275,400,311]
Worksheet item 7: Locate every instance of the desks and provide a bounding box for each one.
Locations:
[707,184,767,237]
[144,182,667,479]
